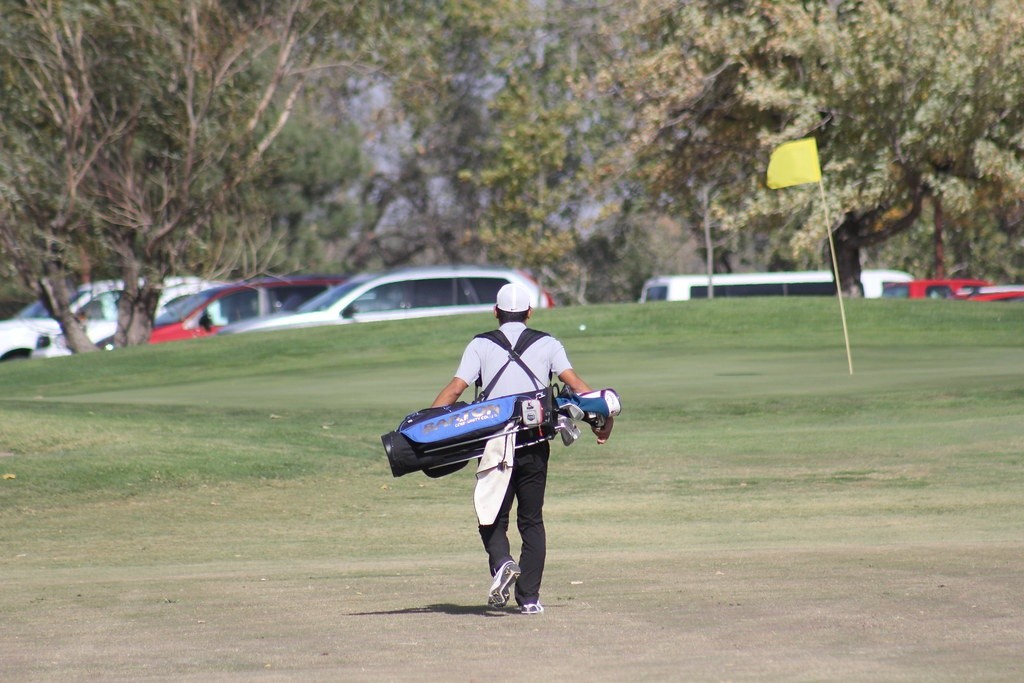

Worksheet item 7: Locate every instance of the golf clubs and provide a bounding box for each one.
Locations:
[556,402,585,447]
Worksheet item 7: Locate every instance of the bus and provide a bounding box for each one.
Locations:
[638,269,939,304]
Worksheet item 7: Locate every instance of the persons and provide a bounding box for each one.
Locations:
[432,283,613,613]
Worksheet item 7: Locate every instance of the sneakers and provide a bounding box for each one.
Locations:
[488,560,521,609]
[520,600,545,614]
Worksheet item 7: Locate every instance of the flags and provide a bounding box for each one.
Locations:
[767,138,821,189]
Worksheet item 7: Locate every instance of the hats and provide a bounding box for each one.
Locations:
[497,284,529,312]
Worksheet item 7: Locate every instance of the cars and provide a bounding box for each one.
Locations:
[884,280,1023,301]
[0,274,342,361]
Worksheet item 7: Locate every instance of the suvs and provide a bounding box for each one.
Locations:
[216,265,555,333]
